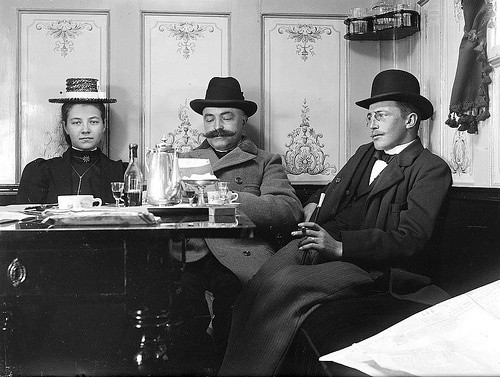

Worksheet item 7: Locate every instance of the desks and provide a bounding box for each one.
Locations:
[0,199,256,377]
[317,278,500,377]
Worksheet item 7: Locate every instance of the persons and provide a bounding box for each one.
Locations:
[167,78,303,377]
[219,69,453,377]
[7,78,150,363]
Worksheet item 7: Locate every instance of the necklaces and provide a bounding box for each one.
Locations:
[72,158,97,196]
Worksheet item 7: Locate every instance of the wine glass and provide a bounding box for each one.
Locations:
[185,184,195,207]
[216,182,228,207]
[110,182,125,208]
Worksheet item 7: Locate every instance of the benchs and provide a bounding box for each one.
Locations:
[0,184,500,377]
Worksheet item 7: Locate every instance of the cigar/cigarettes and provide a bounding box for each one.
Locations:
[301,226,306,230]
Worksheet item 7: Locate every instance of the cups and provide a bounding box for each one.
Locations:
[57,195,102,211]
[208,191,238,204]
[348,7,368,34]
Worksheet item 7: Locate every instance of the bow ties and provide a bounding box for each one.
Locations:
[374,149,396,164]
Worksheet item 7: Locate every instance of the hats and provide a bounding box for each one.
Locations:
[49,77,118,104]
[355,69,434,120]
[190,76,257,117]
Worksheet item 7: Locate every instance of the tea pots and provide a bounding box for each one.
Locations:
[144,139,182,207]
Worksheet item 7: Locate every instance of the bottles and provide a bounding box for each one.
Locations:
[396,4,411,28]
[371,0,396,32]
[123,143,143,207]
